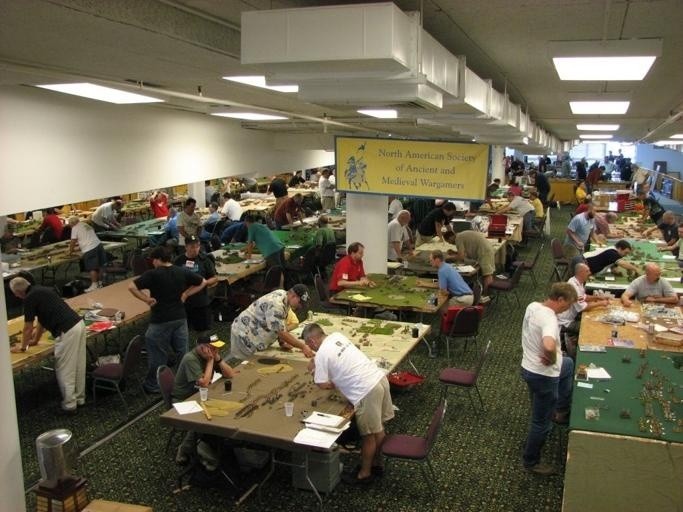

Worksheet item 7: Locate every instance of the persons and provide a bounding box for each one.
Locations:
[300,323,395,484]
[330,153,560,319]
[0,166,347,415]
[520,155,681,474]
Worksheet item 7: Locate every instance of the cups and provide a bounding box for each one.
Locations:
[411,327,418,339]
[432,298,438,306]
[224,380,231,390]
[199,388,208,400]
[283,401,294,417]
[649,324,654,335]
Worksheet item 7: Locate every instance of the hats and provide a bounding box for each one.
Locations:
[291,283,313,304]
[183,234,200,245]
[197,329,225,348]
[527,169,536,176]
[144,245,169,261]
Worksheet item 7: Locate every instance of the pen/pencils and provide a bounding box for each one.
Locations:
[590,397,604,400]
[277,366,284,373]
[317,413,330,418]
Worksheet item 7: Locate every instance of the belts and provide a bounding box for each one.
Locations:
[453,292,475,297]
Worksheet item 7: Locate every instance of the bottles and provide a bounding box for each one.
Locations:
[610,325,618,338]
[576,364,587,380]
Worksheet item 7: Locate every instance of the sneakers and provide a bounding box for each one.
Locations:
[83,283,104,294]
[555,200,561,212]
[52,398,87,417]
[550,409,569,424]
[341,462,386,486]
[527,460,556,477]
